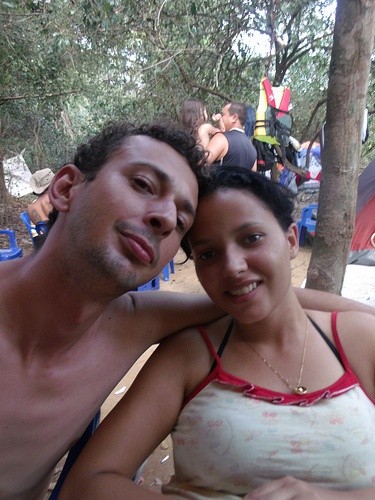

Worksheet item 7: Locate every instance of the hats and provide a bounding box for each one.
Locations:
[31,168,56,194]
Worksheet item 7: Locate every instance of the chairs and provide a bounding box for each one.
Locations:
[0,211,176,291]
[295,204,319,248]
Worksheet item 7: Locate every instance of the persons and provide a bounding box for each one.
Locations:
[179,99,228,153]
[58,164,375,500]
[0,118,375,500]
[197,101,258,172]
[27,167,56,225]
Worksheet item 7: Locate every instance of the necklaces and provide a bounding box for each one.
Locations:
[233,314,309,395]
